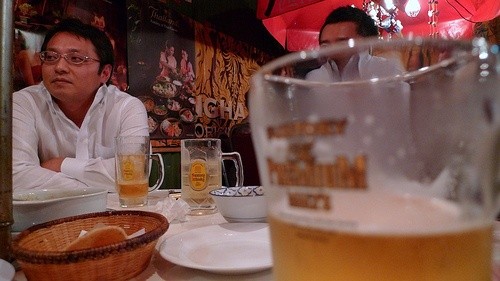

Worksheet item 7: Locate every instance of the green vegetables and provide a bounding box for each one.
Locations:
[158,104,167,110]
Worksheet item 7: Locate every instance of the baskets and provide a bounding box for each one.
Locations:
[7,210,169,281]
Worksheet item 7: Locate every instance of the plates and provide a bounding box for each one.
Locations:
[0,258,15,281]
[159,223,272,276]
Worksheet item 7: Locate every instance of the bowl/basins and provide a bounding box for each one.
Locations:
[209,186,268,223]
[137,80,196,138]
[11,187,109,232]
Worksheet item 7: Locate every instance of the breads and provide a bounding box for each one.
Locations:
[63,226,128,250]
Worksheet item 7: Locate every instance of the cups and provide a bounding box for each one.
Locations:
[180,138,244,216]
[247,38,500,280]
[112,136,164,209]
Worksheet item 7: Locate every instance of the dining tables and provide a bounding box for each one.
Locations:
[0,186,280,281]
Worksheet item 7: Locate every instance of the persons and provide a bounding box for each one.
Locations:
[304,6,418,180]
[157,40,195,83]
[11,18,153,193]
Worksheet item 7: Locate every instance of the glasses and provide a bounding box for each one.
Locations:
[38,50,100,65]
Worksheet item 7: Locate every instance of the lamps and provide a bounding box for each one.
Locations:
[371,0,421,17]
